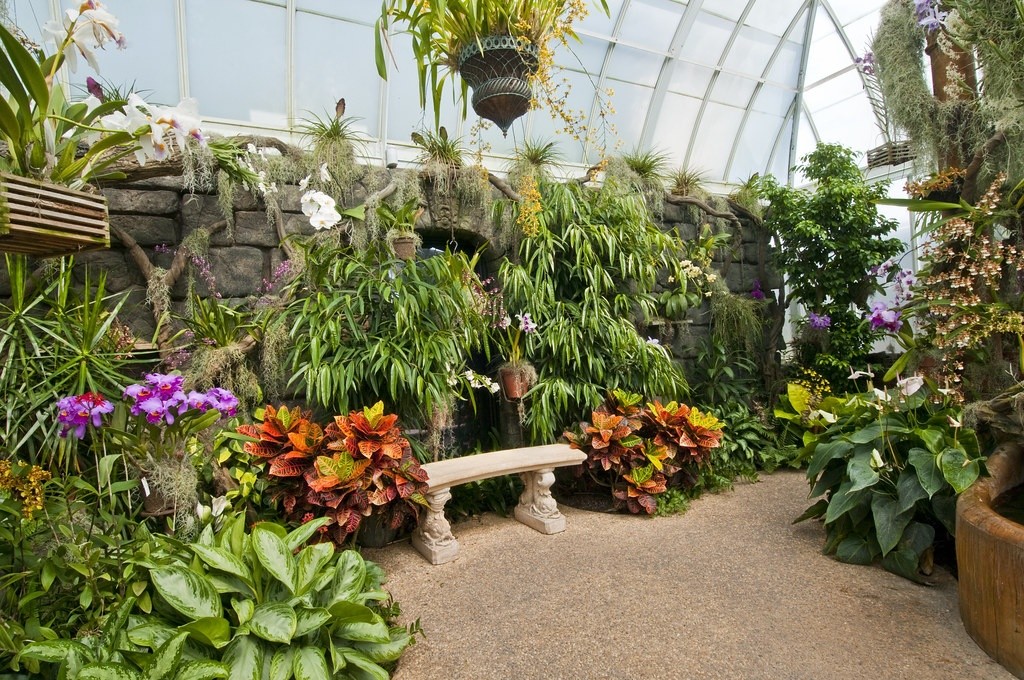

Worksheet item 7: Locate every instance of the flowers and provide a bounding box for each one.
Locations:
[0,460,53,629]
[57,373,240,533]
[0,0,212,191]
[207,139,278,195]
[375,0,623,237]
[299,163,341,231]
[752,280,774,308]
[913,0,983,99]
[497,311,538,366]
[788,165,1024,590]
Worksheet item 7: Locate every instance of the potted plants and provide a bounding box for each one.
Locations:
[377,194,425,262]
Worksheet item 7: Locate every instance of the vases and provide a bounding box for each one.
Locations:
[501,367,529,400]
[458,35,539,139]
[0,172,111,258]
